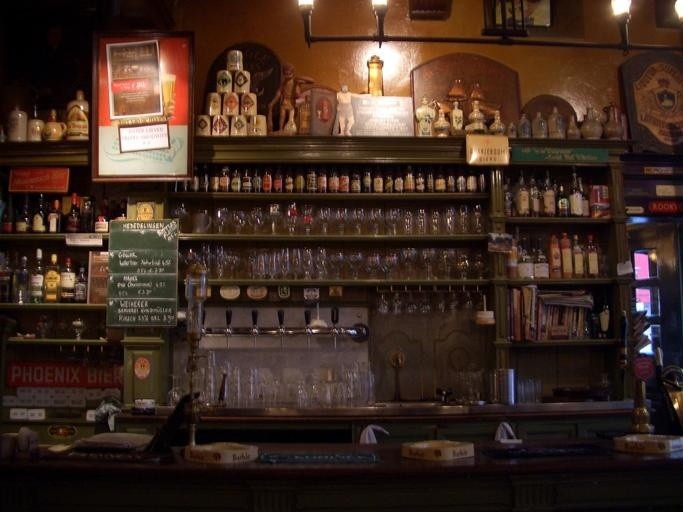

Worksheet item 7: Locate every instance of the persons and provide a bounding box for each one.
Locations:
[337,85,361,136]
[268,62,315,130]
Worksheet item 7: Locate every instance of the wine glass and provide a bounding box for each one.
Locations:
[71,313,85,340]
[36,314,49,339]
[173,204,487,280]
[375,290,487,313]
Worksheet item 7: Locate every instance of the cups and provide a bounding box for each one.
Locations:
[515,377,543,404]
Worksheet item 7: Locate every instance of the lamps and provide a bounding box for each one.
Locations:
[298,1,682,53]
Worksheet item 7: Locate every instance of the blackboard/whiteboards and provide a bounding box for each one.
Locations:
[106,218,179,327]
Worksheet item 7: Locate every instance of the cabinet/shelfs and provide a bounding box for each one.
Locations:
[490,139,624,401]
[166,134,493,287]
[0,142,125,353]
[349,407,516,444]
[516,404,634,442]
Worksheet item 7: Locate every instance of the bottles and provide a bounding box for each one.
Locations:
[5,90,89,140]
[503,171,591,217]
[171,170,488,195]
[595,293,611,338]
[0,186,93,235]
[168,348,377,409]
[508,228,601,278]
[0,250,87,304]
[414,96,624,139]
[195,49,267,136]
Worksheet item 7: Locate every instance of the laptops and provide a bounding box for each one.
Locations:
[74,392,200,453]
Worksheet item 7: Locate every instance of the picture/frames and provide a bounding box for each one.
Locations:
[89,26,197,185]
